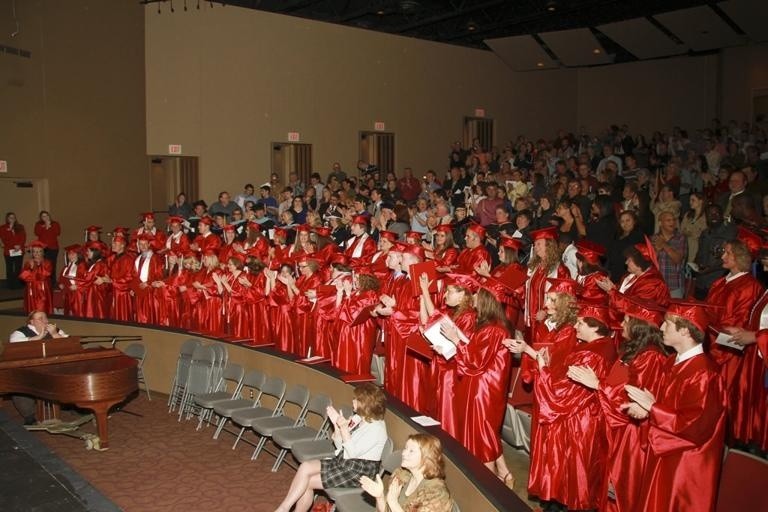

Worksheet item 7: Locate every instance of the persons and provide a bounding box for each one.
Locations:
[1,116,767,511]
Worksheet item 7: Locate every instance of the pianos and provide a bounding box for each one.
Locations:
[0,337,138,451]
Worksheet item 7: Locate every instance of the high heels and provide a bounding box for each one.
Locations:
[497,472,516,491]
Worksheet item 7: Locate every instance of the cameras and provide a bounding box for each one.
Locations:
[465,186,470,193]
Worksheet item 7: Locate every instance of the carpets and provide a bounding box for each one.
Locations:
[0,388,297,512]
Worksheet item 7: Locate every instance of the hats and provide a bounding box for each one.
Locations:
[380,218,520,250]
[355,194,369,203]
[573,242,604,266]
[224,213,371,274]
[379,243,520,305]
[637,235,660,268]
[249,203,266,212]
[27,240,47,247]
[63,226,128,253]
[543,276,726,335]
[192,200,208,207]
[453,201,470,208]
[137,210,220,257]
[527,224,560,241]
